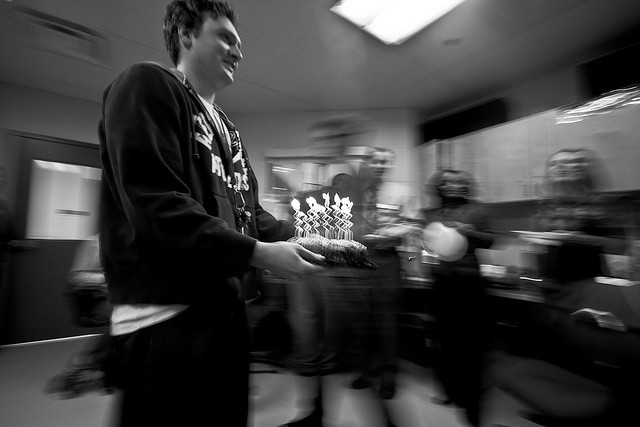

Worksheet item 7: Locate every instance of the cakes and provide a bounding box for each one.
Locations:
[288,236,369,265]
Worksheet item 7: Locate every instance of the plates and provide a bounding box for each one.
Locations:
[509,229,574,246]
[420,220,470,262]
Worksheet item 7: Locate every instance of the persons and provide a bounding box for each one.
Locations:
[418,168,495,427]
[529,145,627,421]
[96,1,326,426]
[311,139,413,403]
